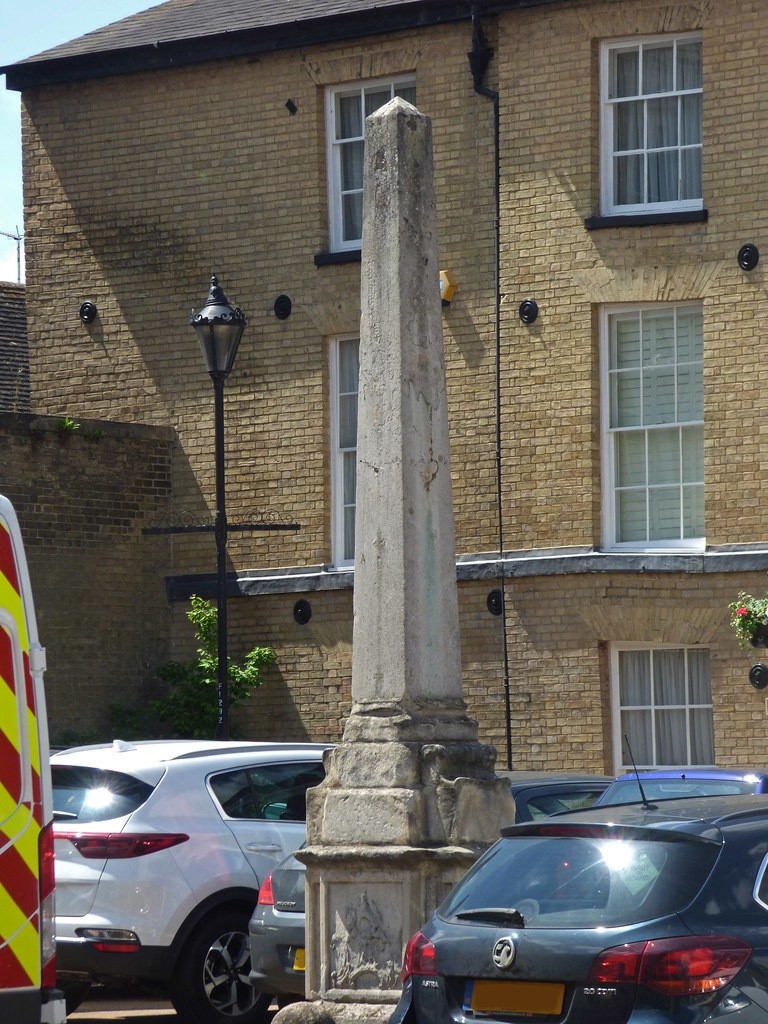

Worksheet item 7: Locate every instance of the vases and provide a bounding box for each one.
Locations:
[750,625,768,649]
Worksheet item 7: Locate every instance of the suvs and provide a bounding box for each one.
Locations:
[388,793,768,1024]
[49,739,342,1024]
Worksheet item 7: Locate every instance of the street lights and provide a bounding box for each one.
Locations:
[189,275,246,741]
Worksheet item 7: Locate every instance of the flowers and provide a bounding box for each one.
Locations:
[727,591,768,659]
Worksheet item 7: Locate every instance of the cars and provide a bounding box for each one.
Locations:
[249,771,616,1012]
[593,769,768,812]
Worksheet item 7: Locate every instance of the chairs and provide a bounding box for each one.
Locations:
[280,774,322,820]
